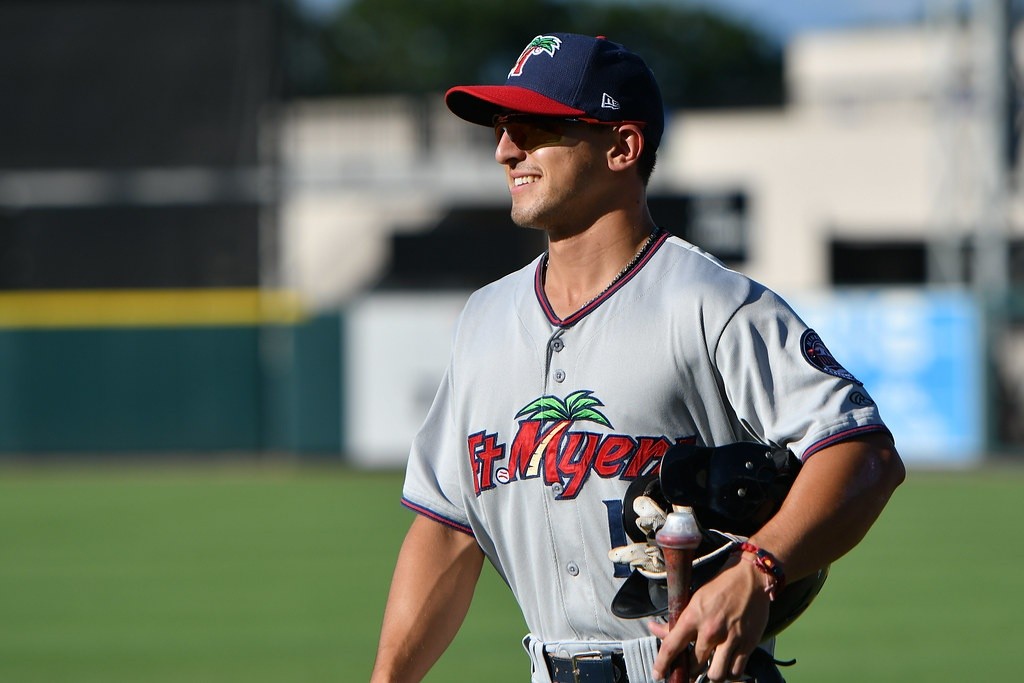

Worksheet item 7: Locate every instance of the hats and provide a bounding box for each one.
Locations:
[445,32,664,147]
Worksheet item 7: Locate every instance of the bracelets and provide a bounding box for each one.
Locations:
[726,540,786,605]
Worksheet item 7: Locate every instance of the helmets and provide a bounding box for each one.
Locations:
[612,441,830,645]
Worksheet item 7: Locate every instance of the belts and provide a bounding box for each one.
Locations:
[545,653,628,683]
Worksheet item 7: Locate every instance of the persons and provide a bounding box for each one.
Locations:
[370,35,906,683]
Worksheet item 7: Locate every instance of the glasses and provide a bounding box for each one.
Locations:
[492,112,646,150]
